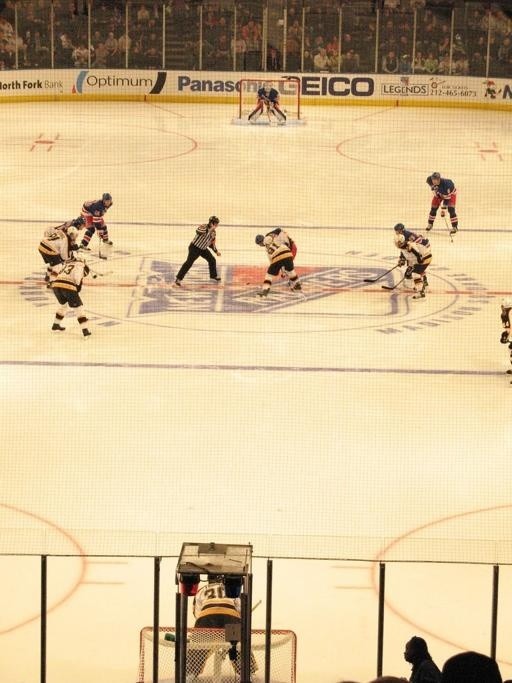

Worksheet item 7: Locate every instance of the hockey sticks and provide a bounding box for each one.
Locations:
[165,601,261,643]
[364,263,400,283]
[382,268,416,290]
[438,196,453,243]
[89,270,113,278]
[267,112,277,125]
[99,235,107,259]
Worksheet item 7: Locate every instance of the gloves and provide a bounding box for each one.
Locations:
[441,210,445,216]
[399,260,405,266]
[404,267,414,278]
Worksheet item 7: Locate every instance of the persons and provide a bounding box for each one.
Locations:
[38,192,114,337]
[265,228,298,279]
[257,234,304,297]
[247,82,287,126]
[393,233,433,300]
[425,171,459,236]
[500,295,512,383]
[393,222,431,290]
[185,575,259,683]
[0,0,511,79]
[173,215,222,287]
[334,635,512,683]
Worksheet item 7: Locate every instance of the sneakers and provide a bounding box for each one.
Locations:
[210,276,221,280]
[175,280,181,286]
[413,291,425,298]
[426,224,433,230]
[257,288,270,295]
[103,239,112,244]
[451,226,458,233]
[79,244,90,250]
[53,324,65,330]
[83,329,91,335]
[289,282,302,290]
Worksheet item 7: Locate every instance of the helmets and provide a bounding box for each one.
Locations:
[103,193,111,200]
[67,226,78,238]
[394,224,404,230]
[256,235,262,243]
[73,251,85,261]
[431,172,440,178]
[210,216,219,223]
[263,236,272,245]
[74,217,85,226]
[394,234,405,247]
